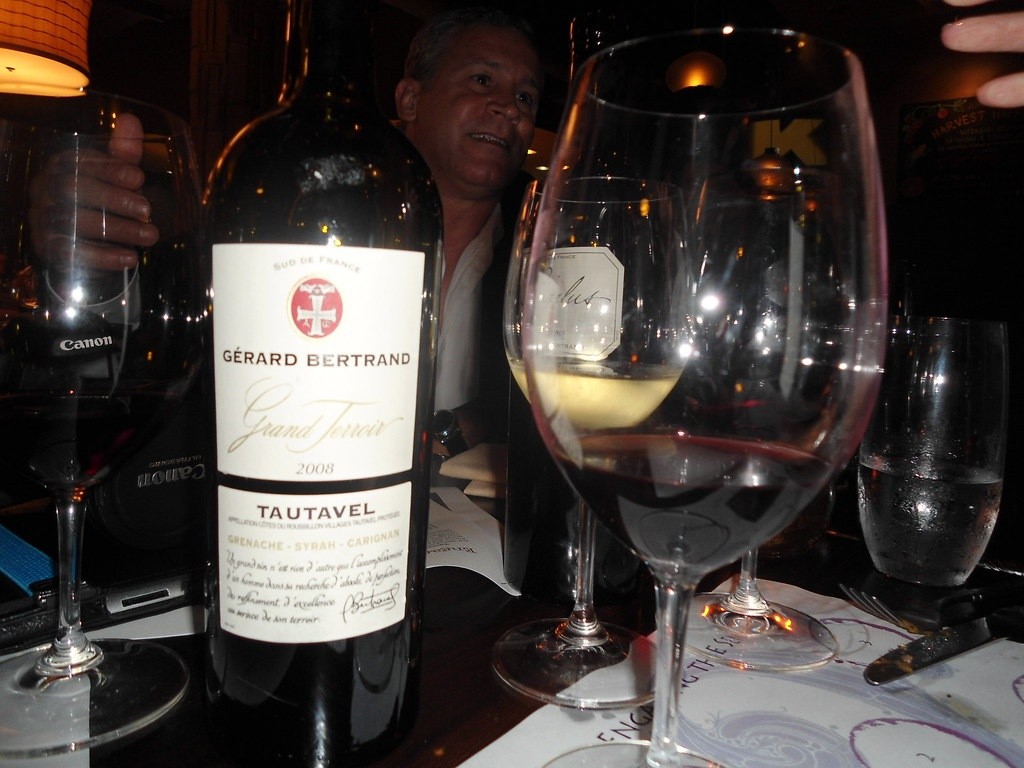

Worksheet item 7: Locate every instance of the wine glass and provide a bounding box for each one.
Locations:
[0,97,205,758]
[489,19,890,768]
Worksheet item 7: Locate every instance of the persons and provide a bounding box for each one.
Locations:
[0,1,1023,501]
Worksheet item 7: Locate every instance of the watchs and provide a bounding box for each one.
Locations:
[429,408,467,462]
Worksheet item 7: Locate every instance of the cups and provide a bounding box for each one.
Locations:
[856,314,1005,587]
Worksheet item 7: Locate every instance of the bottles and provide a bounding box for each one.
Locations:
[205,0,446,768]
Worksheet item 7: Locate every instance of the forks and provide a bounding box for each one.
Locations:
[837,583,982,636]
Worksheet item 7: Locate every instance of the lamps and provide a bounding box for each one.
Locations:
[0,0,92,96]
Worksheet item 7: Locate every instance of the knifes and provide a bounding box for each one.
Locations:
[865,604,1024,685]
